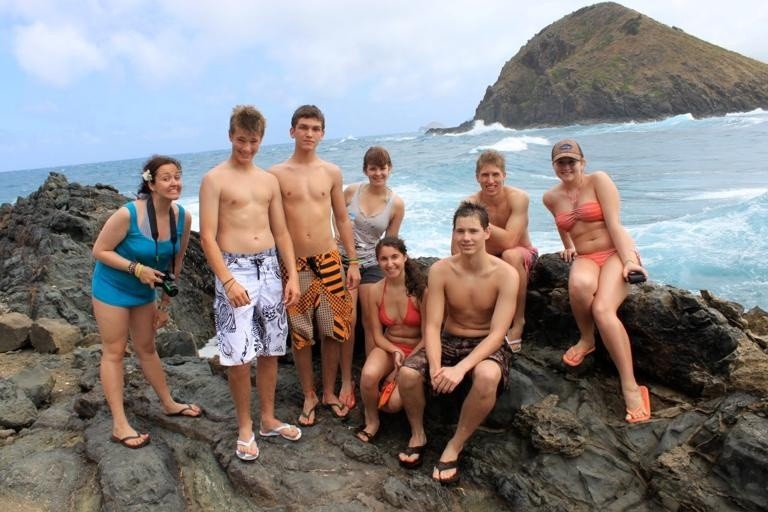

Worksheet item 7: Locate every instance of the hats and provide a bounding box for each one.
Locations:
[552,140,583,163]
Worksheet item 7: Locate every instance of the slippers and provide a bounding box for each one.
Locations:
[355,429,378,442]
[505,329,523,354]
[322,400,350,418]
[562,344,596,367]
[433,456,461,484]
[625,385,651,424]
[111,433,150,449]
[167,404,202,418]
[236,433,260,462]
[399,441,426,469]
[338,380,357,409]
[259,422,303,442]
[298,400,320,427]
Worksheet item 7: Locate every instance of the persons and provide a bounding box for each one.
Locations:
[197,99,302,463]
[352,234,431,443]
[88,154,203,449]
[395,200,520,486]
[542,139,652,423]
[265,103,363,431]
[331,146,405,410]
[447,149,539,356]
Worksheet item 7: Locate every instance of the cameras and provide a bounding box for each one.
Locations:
[154,272,179,297]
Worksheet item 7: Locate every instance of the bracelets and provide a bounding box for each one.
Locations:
[157,305,169,313]
[134,262,145,278]
[620,258,636,265]
[348,257,360,268]
[127,260,137,277]
[220,277,237,295]
